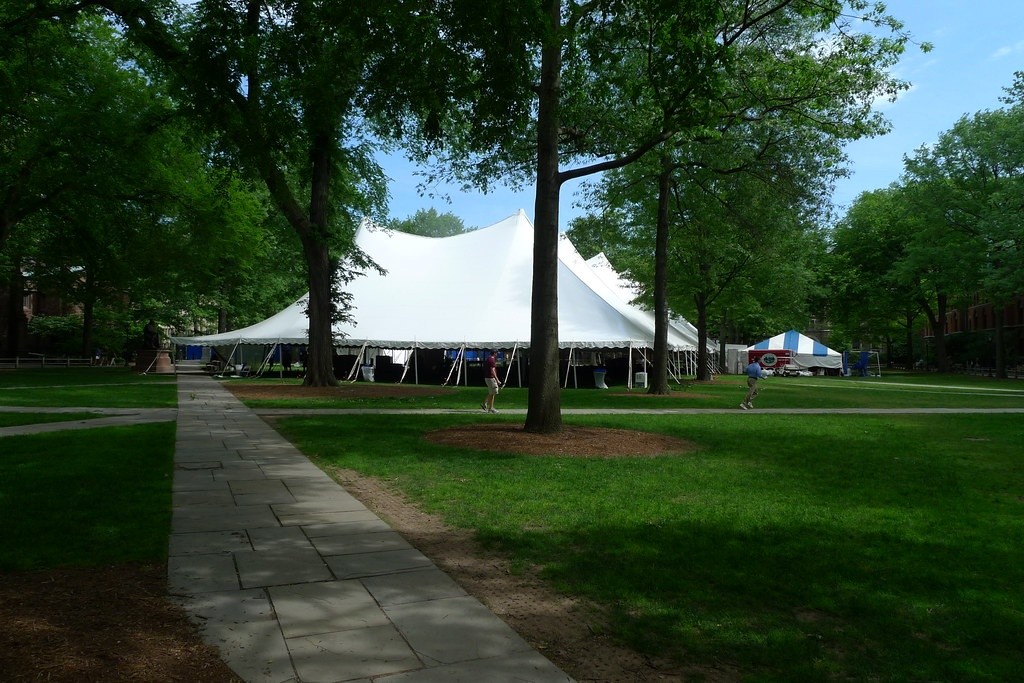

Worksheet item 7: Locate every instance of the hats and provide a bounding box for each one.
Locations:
[491,347,500,353]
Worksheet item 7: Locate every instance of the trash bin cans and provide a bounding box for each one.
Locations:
[635,372,648,388]
[593,369,607,388]
[362,366,375,382]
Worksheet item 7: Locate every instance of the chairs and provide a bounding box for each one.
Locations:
[241,365,252,378]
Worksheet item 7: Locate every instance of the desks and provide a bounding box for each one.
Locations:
[361,366,377,382]
[592,370,608,389]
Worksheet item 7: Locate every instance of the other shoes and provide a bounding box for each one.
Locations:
[488,408,499,414]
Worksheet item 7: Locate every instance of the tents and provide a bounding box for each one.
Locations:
[168,208,720,390]
[745,330,844,376]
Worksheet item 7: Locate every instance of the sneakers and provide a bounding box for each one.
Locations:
[748,402,753,408]
[739,404,747,410]
[480,400,487,411]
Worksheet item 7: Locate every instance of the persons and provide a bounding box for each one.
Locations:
[481,349,502,413]
[739,356,766,410]
